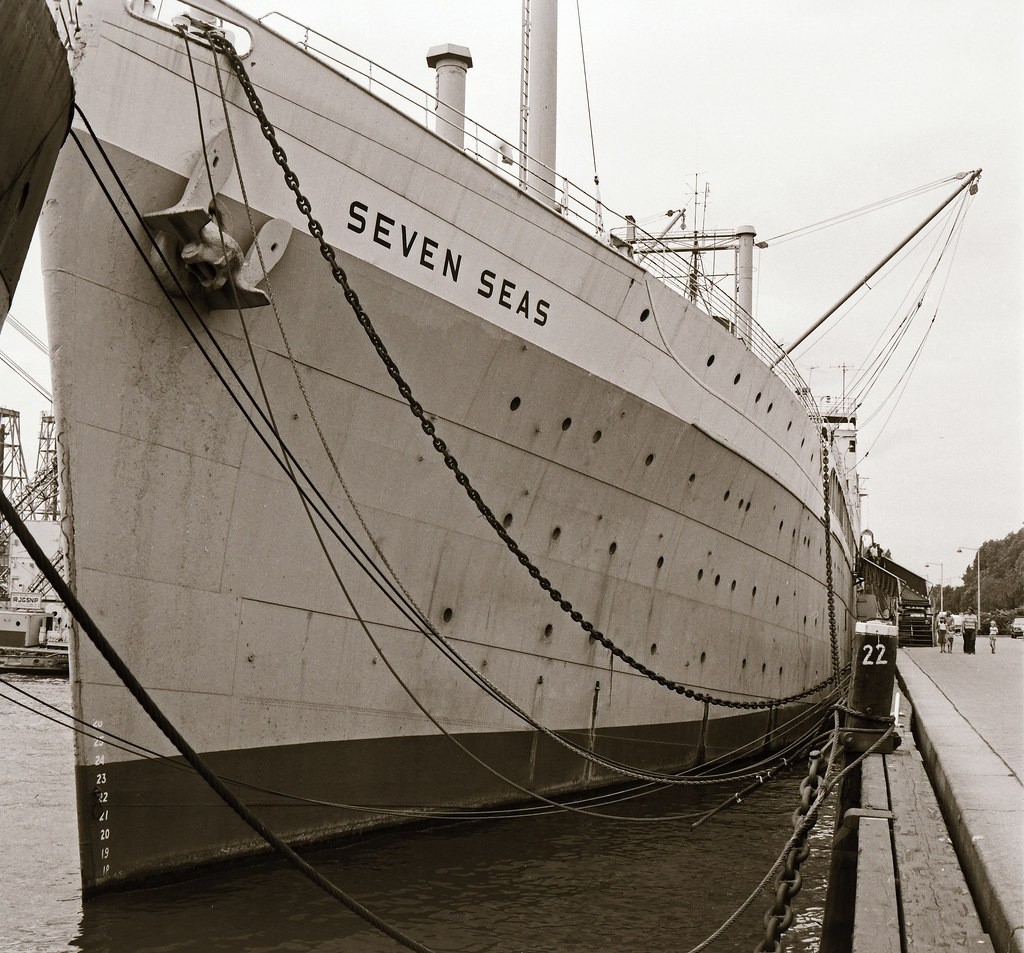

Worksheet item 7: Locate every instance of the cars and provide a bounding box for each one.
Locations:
[1010,617,1024,638]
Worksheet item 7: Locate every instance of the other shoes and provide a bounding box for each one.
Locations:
[973,652,975,654]
[943,650,946,653]
[992,651,995,654]
[940,652,942,653]
[968,652,971,654]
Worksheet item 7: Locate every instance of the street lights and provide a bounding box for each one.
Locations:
[956,547,980,636]
[926,563,943,612]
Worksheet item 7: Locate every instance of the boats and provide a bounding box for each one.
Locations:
[0,0,982,903]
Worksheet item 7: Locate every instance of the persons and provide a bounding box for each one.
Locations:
[989,620,999,653]
[867,543,883,566]
[937,606,978,654]
